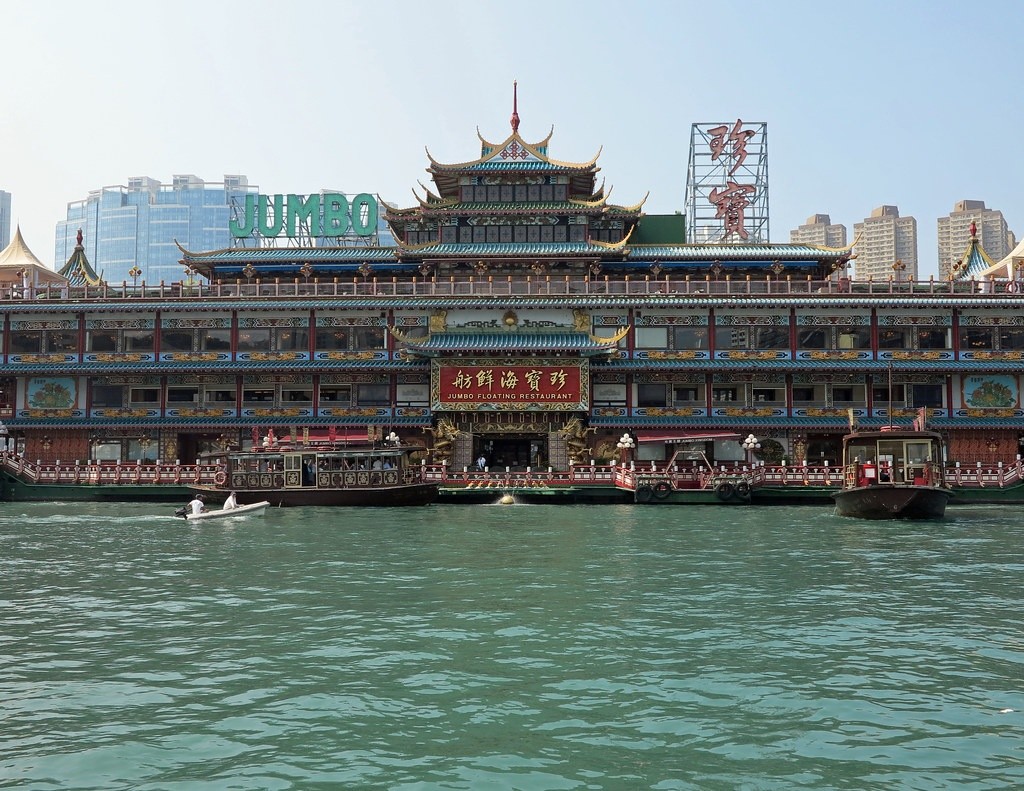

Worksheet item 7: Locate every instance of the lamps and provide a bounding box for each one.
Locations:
[40,436,53,446]
[987,437,1000,449]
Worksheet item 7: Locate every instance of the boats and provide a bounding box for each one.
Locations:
[174,501,272,526]
[831,361,956,522]
[185,400,440,506]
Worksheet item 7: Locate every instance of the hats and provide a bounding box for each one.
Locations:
[196,494,203,499]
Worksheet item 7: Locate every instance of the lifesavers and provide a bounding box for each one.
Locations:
[652,481,672,499]
[735,482,752,499]
[715,481,734,500]
[214,471,228,485]
[635,485,653,503]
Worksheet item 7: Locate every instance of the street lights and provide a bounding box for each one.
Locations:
[530,260,546,293]
[88,435,102,464]
[417,261,431,294]
[648,260,664,292]
[138,434,152,470]
[740,434,761,470]
[16,261,380,299]
[708,260,724,293]
[832,260,845,285]
[986,437,1000,470]
[1014,261,1024,293]
[769,259,785,293]
[216,433,231,464]
[617,433,636,468]
[40,436,54,469]
[472,261,488,294]
[891,260,906,293]
[589,261,604,292]
[793,434,808,472]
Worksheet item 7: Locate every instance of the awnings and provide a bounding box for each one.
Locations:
[277,429,380,445]
[637,430,741,445]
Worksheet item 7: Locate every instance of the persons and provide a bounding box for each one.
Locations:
[372,457,391,469]
[260,459,299,472]
[303,459,316,485]
[223,492,242,509]
[188,494,209,515]
[858,450,865,478]
[477,454,486,471]
[318,459,368,470]
